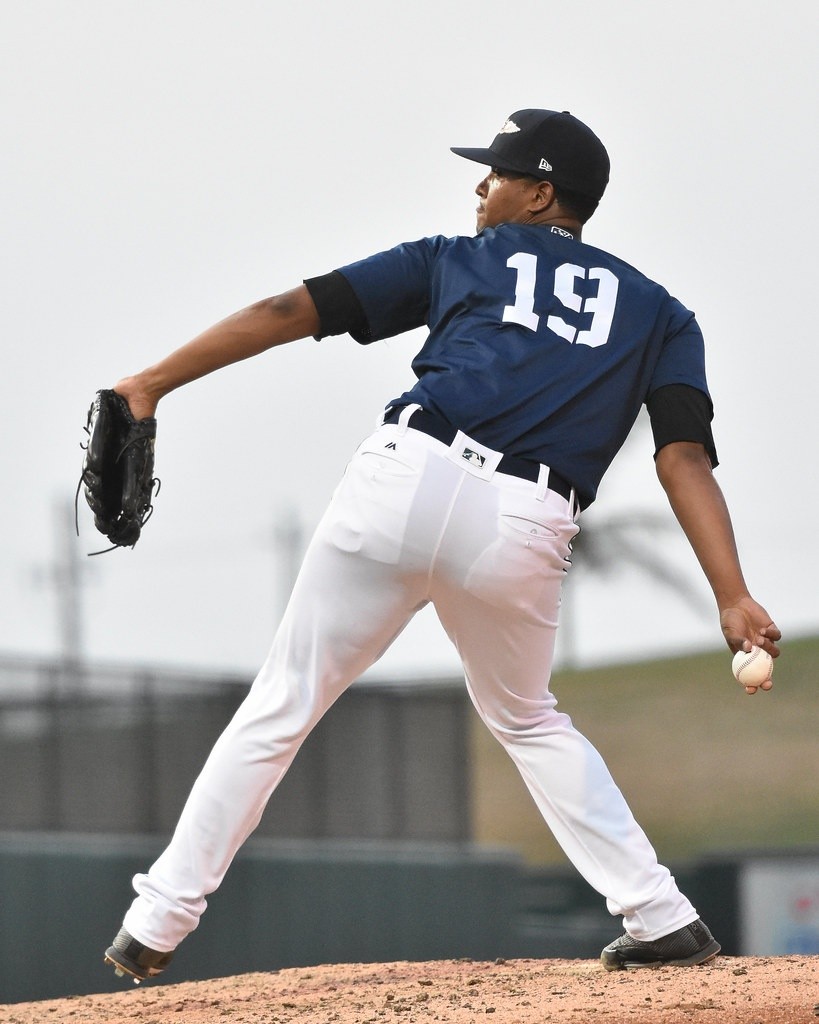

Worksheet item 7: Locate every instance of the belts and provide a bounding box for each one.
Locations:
[382,404,579,517]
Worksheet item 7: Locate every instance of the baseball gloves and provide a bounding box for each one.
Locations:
[76,387,159,552]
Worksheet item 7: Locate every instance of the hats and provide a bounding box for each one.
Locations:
[450,108,610,201]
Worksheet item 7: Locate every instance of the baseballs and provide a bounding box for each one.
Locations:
[732,639,778,696]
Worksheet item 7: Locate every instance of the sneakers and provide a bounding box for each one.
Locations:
[600,917,721,972]
[104,927,172,984]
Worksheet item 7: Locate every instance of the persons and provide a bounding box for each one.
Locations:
[94,104,783,986]
